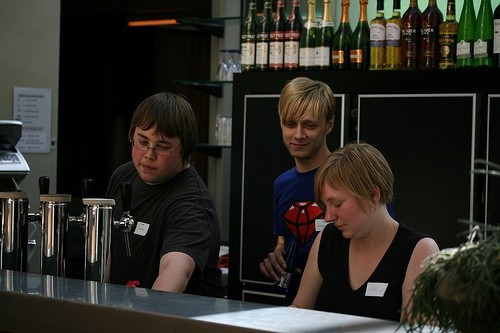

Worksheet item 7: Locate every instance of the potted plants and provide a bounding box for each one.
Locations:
[394,219,500,333]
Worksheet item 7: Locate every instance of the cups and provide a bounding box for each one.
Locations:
[214,116,232,146]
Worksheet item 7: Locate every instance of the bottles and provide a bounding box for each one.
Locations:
[401,0,422,70]
[493,4,500,69]
[315,0,335,71]
[269,0,287,71]
[300,0,319,70]
[272,236,299,295]
[240,0,259,71]
[387,0,401,70]
[333,0,353,71]
[456,0,477,69]
[256,0,274,71]
[284,0,303,70]
[475,0,494,69]
[419,0,443,70]
[370,0,387,70]
[439,0,459,69]
[349,0,370,71]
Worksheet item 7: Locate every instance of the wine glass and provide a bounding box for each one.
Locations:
[217,50,228,81]
[228,50,241,81]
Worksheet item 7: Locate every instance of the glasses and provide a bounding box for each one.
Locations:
[129,135,182,157]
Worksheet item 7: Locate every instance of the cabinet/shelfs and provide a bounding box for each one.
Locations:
[172,18,225,157]
[226,64,500,327]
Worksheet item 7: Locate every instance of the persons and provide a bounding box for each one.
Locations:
[102,91,222,295]
[289,142,441,327]
[258,77,395,307]
